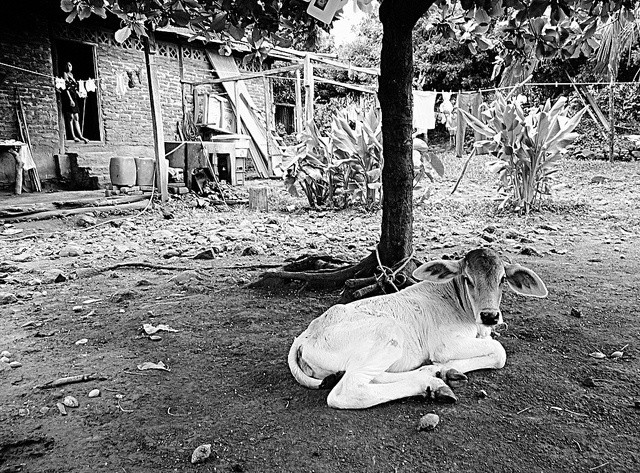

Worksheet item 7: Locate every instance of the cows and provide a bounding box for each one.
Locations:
[288,247,549,409]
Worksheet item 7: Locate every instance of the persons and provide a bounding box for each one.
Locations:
[62,61,90,143]
